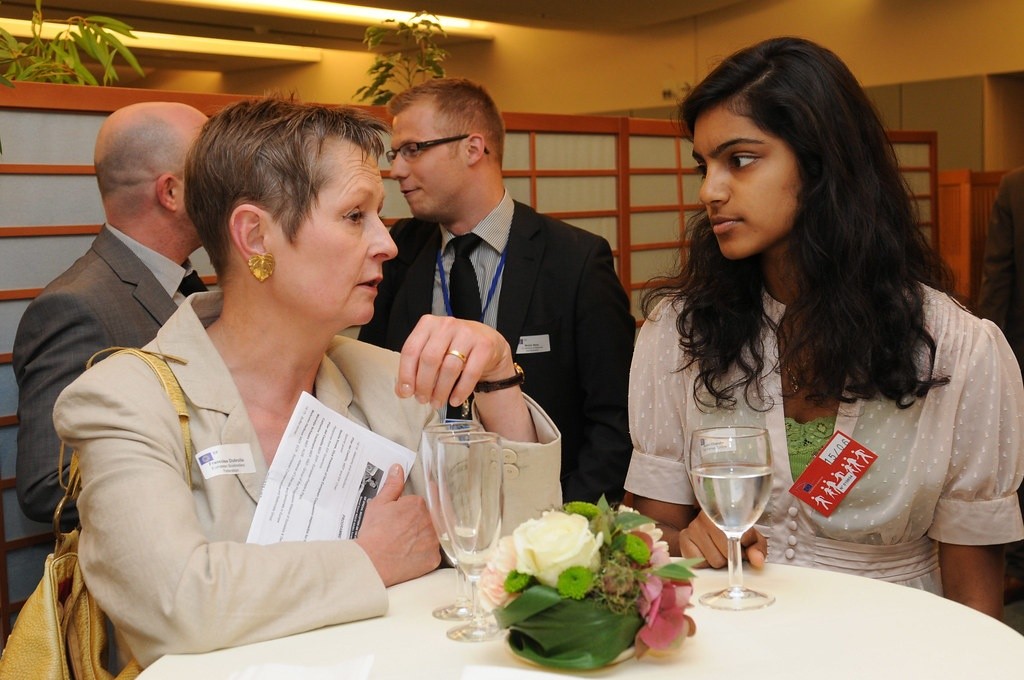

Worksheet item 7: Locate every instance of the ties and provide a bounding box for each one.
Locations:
[444,233,483,423]
[178,270,210,300]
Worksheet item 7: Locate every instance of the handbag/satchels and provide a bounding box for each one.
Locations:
[0,345,193,680]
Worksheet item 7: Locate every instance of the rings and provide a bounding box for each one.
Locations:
[446,350,467,362]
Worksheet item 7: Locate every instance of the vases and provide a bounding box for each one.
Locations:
[605,614,643,667]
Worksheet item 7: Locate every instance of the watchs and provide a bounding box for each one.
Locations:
[474,363,525,393]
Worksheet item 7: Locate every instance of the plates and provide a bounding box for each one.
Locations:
[482,562,519,608]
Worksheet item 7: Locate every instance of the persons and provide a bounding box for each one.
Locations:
[363,471,377,489]
[13,103,209,542]
[624,36,1024,620]
[52,94,562,670]
[982,166,1024,379]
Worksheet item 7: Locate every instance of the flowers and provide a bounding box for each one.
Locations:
[478,494,701,672]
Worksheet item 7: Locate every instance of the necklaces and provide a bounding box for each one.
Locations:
[359,78,638,506]
[780,348,815,393]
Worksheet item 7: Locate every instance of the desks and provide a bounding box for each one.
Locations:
[132,555,1024,680]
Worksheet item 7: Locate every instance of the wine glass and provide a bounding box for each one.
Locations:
[690,427,776,611]
[421,423,484,621]
[437,432,506,643]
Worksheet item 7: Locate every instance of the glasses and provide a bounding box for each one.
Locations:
[386,133,490,167]
